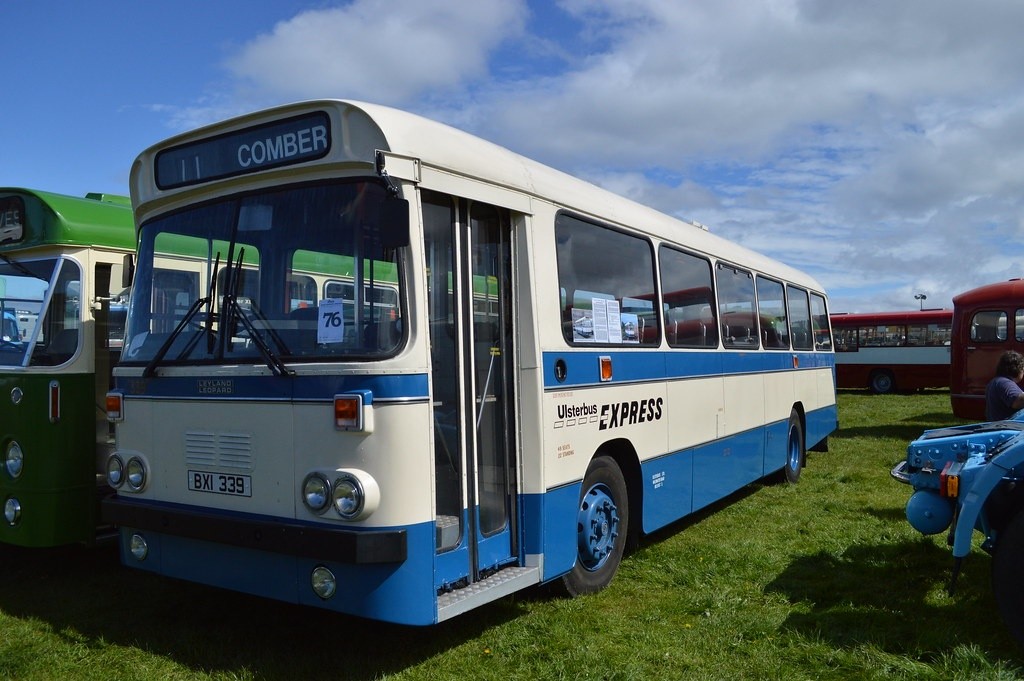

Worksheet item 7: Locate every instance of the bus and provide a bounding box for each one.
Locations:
[0,187,670,609]
[950,278,1024,421]
[107,101,838,627]
[826,309,954,394]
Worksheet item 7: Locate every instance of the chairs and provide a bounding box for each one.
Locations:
[44,329,79,364]
[273,307,324,352]
[360,321,499,410]
[634,316,816,344]
